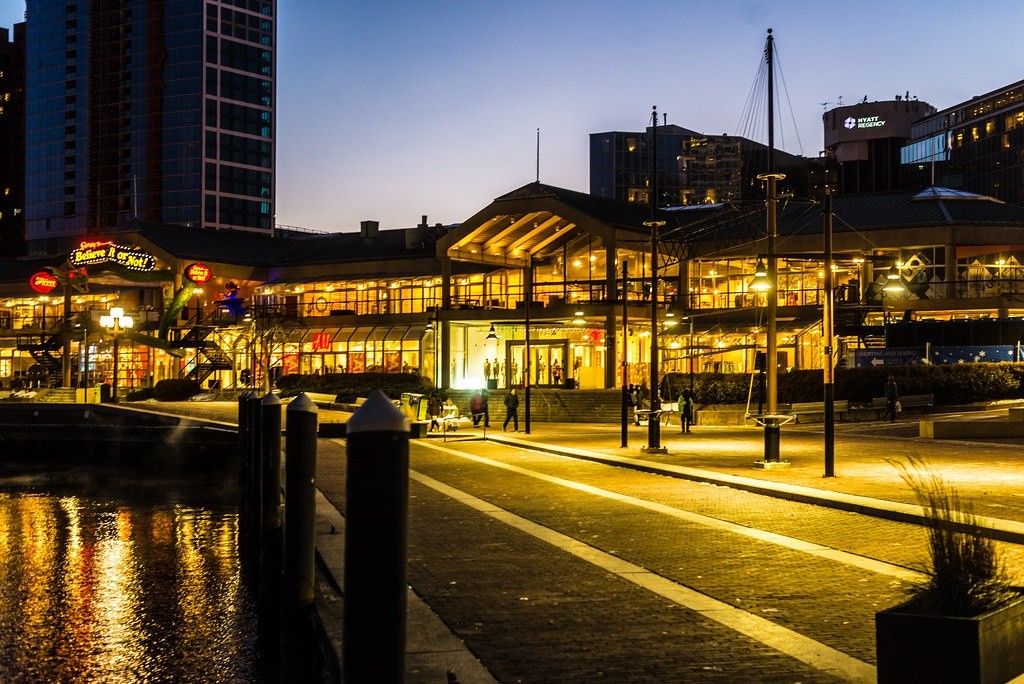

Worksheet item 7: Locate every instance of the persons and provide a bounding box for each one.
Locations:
[880,375,898,423]
[427,388,519,432]
[452,355,560,390]
[626,383,694,433]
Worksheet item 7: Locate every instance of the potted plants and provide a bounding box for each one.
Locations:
[873,447,1024,683]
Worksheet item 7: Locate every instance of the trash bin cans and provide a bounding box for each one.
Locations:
[398,392,412,417]
[409,394,428,422]
[93,382,110,404]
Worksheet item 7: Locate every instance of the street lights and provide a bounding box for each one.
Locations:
[99,307,134,406]
[485,292,565,436]
[746,195,905,480]
[424,303,440,393]
[569,261,682,450]
[662,313,697,426]
